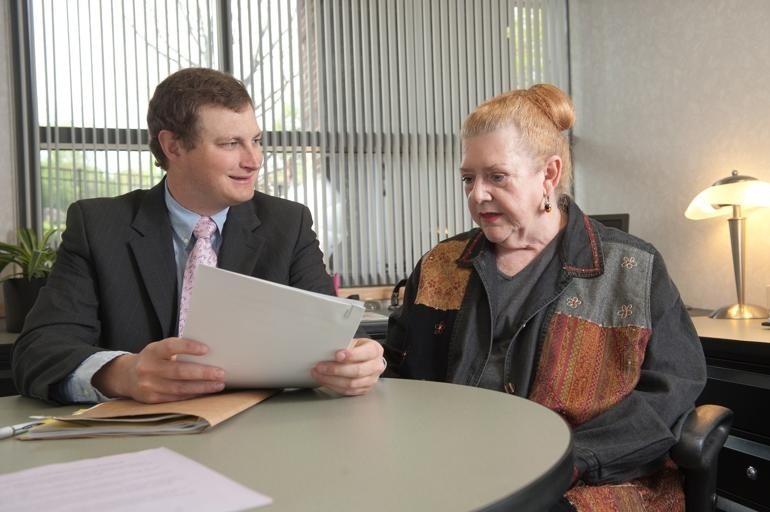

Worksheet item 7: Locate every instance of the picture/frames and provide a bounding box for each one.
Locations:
[356,307,770,512]
[0,378,571,512]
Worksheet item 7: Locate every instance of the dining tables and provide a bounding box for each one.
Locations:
[0,228,59,333]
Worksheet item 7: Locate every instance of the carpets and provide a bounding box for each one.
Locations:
[179,216,217,337]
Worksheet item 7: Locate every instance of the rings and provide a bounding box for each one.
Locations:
[0,420,44,440]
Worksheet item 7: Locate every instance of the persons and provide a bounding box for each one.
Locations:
[11,67,387,406]
[375,81,712,512]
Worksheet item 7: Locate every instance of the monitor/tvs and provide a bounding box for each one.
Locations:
[14,385,291,445]
[360,308,390,323]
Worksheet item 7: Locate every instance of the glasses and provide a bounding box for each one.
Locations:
[685,171,770,320]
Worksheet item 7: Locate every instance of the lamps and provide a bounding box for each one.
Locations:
[670,404,737,512]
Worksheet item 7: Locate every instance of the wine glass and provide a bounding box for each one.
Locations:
[380,356,387,374]
